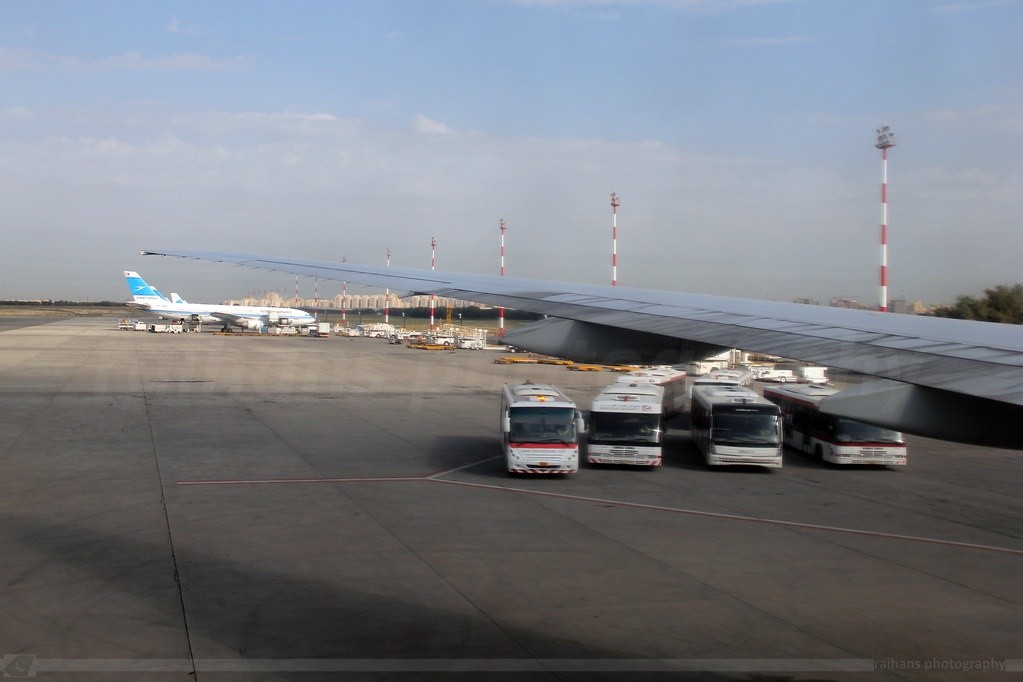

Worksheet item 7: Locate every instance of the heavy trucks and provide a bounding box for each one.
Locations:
[731,362,792,383]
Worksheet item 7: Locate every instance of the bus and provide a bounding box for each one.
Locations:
[587,383,666,468]
[687,358,728,375]
[617,366,686,422]
[500,380,585,475]
[118,318,489,354]
[763,383,907,468]
[694,368,753,391]
[690,385,782,468]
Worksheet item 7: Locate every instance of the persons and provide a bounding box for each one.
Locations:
[639,424,654,433]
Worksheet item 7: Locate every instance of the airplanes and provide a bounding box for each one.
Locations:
[123,270,316,333]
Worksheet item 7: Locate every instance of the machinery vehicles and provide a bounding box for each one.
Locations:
[493,345,643,373]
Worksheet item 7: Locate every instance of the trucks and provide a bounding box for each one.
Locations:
[797,366,829,385]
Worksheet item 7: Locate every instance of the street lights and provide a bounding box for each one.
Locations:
[874,126,897,311]
[385,247,390,323]
[431,237,435,330]
[500,218,505,339]
[610,192,620,286]
[342,256,346,324]
